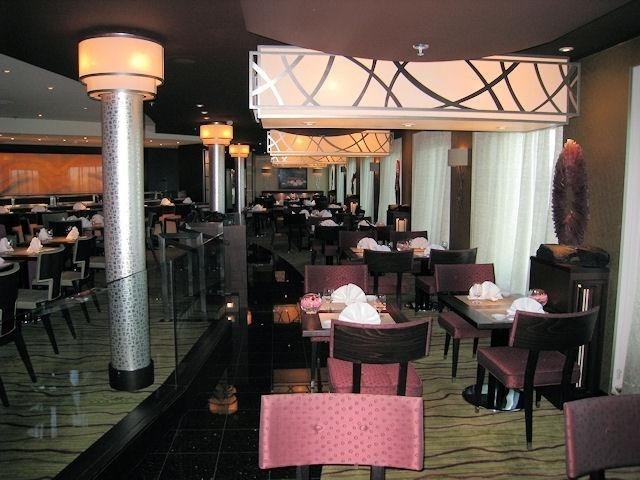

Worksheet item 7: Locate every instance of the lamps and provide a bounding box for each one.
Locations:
[265,130,394,171]
[206,384,237,414]
[227,292,245,327]
[228,143,250,158]
[77,29,166,100]
[274,303,302,327]
[448,148,469,209]
[198,120,234,147]
[270,359,326,391]
[249,40,585,132]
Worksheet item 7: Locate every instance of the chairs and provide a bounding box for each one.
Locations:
[474,306,600,449]
[325,316,432,398]
[241,192,477,314]
[435,263,496,382]
[259,392,425,480]
[563,394,639,480]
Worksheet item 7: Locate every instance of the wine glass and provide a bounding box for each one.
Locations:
[323,289,333,310]
[374,295,385,319]
[529,289,547,306]
[441,242,448,249]
[397,241,408,251]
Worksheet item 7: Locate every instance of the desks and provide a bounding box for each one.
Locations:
[529,253,610,412]
